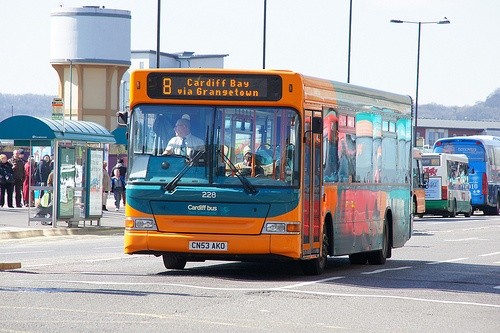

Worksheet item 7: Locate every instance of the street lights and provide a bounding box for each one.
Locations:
[389,19,452,148]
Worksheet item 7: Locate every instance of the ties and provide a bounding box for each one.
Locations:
[180,138,187,157]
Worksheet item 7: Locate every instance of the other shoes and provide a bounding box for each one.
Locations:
[25,201,35,207]
[115,208,119,211]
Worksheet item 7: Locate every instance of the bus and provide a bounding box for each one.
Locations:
[115,68,414,276]
[420,152,472,218]
[412,148,426,219]
[432,135,500,216]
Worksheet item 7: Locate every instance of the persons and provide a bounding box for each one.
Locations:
[102,158,127,212]
[0,149,54,226]
[161,119,205,166]
[233,145,298,183]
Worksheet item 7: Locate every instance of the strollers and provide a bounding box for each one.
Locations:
[33,191,53,226]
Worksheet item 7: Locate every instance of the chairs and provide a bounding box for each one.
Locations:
[255,143,295,175]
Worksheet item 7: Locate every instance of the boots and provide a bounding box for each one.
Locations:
[102,205,108,211]
